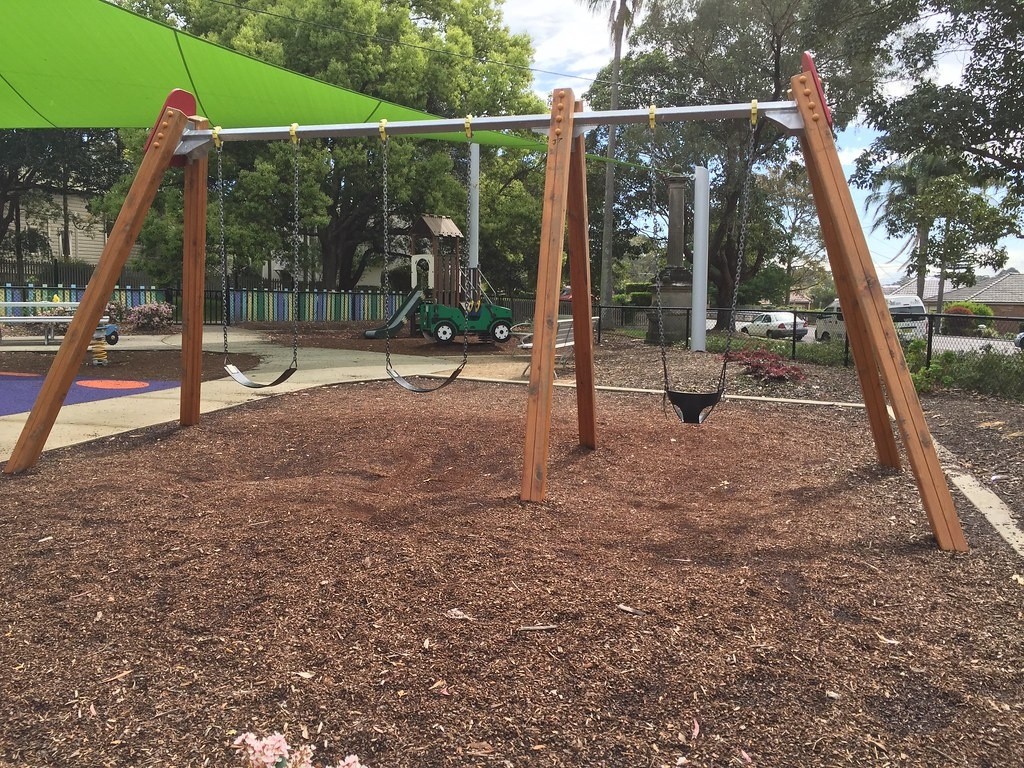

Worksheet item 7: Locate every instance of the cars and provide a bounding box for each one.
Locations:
[559,286,595,303]
[738,312,808,342]
[1013,331,1024,350]
[418,300,514,343]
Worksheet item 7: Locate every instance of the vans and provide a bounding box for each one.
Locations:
[815,294,929,346]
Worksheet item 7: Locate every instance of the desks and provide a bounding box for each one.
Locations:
[0,302,110,341]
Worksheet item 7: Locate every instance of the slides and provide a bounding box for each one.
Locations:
[361,283,426,341]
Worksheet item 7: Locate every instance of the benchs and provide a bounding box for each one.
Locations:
[521,316,600,380]
[0,316,110,346]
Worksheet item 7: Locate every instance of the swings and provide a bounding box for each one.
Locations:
[379,138,473,393]
[649,128,756,425]
[215,144,299,389]
